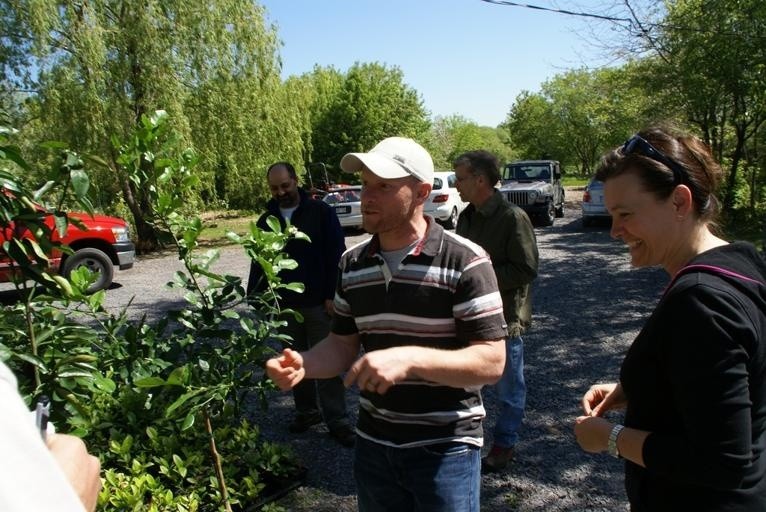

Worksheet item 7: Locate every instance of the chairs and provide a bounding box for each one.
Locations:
[540,169,549,177]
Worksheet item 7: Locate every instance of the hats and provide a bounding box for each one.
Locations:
[340,137,434,186]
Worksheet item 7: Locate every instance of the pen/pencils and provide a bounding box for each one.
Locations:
[36,395,51,444]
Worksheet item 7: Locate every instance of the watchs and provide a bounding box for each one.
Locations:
[608,423,626,459]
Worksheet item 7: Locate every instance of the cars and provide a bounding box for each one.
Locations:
[582,174,613,220]
[322,184,365,230]
[422,170,471,229]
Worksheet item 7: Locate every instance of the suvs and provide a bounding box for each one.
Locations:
[497,161,566,225]
[1,185,136,294]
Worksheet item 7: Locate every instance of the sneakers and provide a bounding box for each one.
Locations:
[296,423,329,434]
[332,428,353,445]
[482,448,511,474]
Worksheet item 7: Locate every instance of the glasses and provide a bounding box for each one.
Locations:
[455,174,480,182]
[622,135,684,182]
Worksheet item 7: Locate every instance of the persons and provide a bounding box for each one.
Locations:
[0,359,102,512]
[245,162,350,444]
[263,135,511,512]
[453,148,539,477]
[570,123,766,511]
